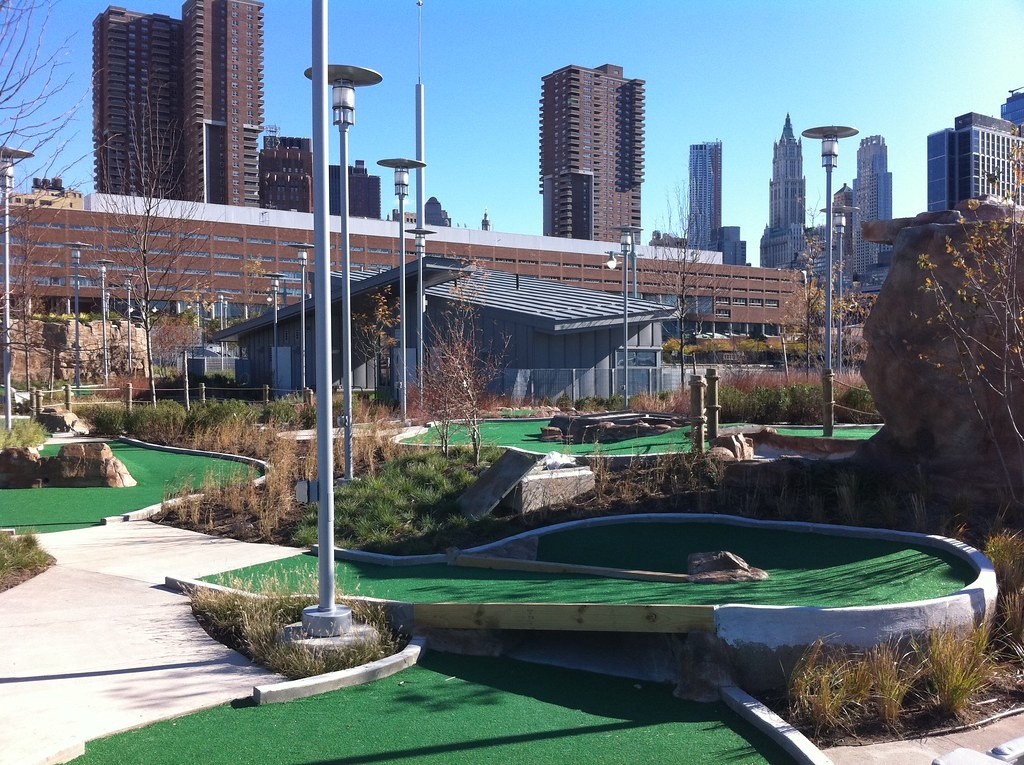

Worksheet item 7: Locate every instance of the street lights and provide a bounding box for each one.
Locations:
[819,201,861,373]
[801,125,861,436]
[92,258,116,387]
[405,228,437,409]
[608,223,645,411]
[604,231,644,300]
[264,270,286,399]
[288,241,316,402]
[304,63,383,479]
[221,296,233,357]
[377,157,426,422]
[62,240,92,394]
[0,145,36,430]
[215,289,230,371]
[120,273,140,375]
